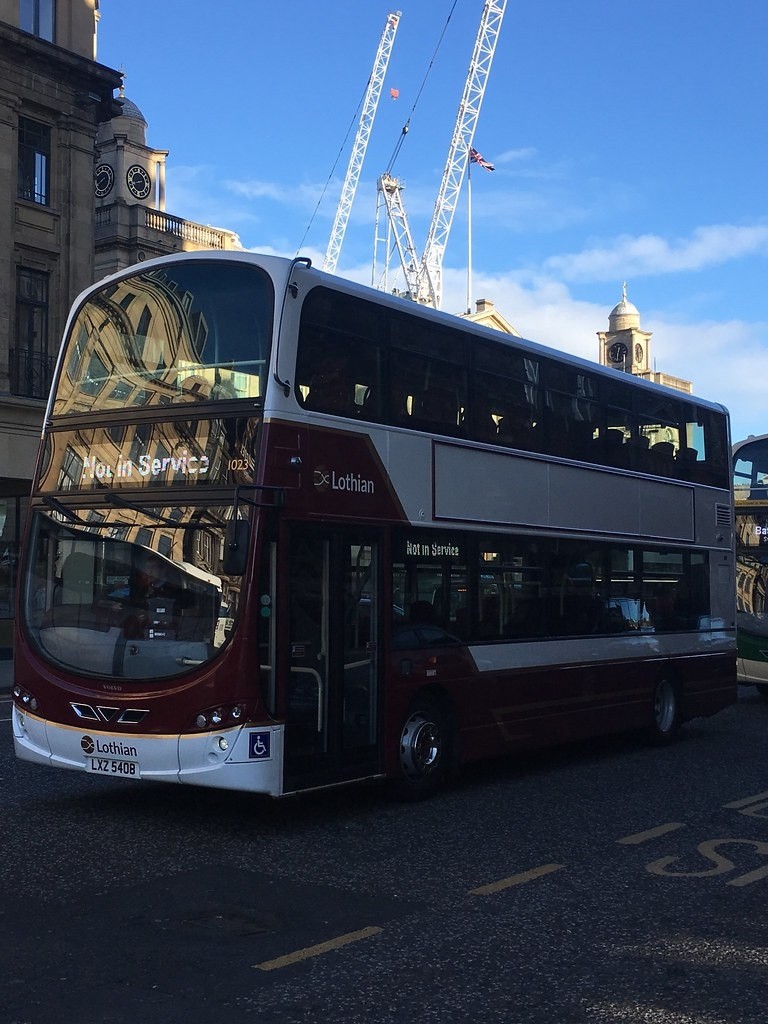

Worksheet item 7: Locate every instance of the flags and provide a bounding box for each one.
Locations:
[469,147,495,172]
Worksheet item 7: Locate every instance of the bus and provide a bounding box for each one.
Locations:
[690,433,768,697]
[11,250,743,811]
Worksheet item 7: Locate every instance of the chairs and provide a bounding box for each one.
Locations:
[411,385,458,425]
[460,400,498,434]
[362,377,409,422]
[651,442,676,462]
[627,434,650,450]
[136,552,182,617]
[674,447,698,467]
[304,358,355,413]
[598,429,624,449]
[497,413,533,440]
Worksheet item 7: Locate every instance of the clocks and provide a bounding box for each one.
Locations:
[93,163,115,199]
[635,343,643,363]
[126,163,151,200]
[608,342,628,363]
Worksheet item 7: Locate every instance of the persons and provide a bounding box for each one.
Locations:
[142,556,175,598]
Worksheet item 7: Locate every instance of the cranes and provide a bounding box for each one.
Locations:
[296,10,406,276]
[370,0,510,314]
